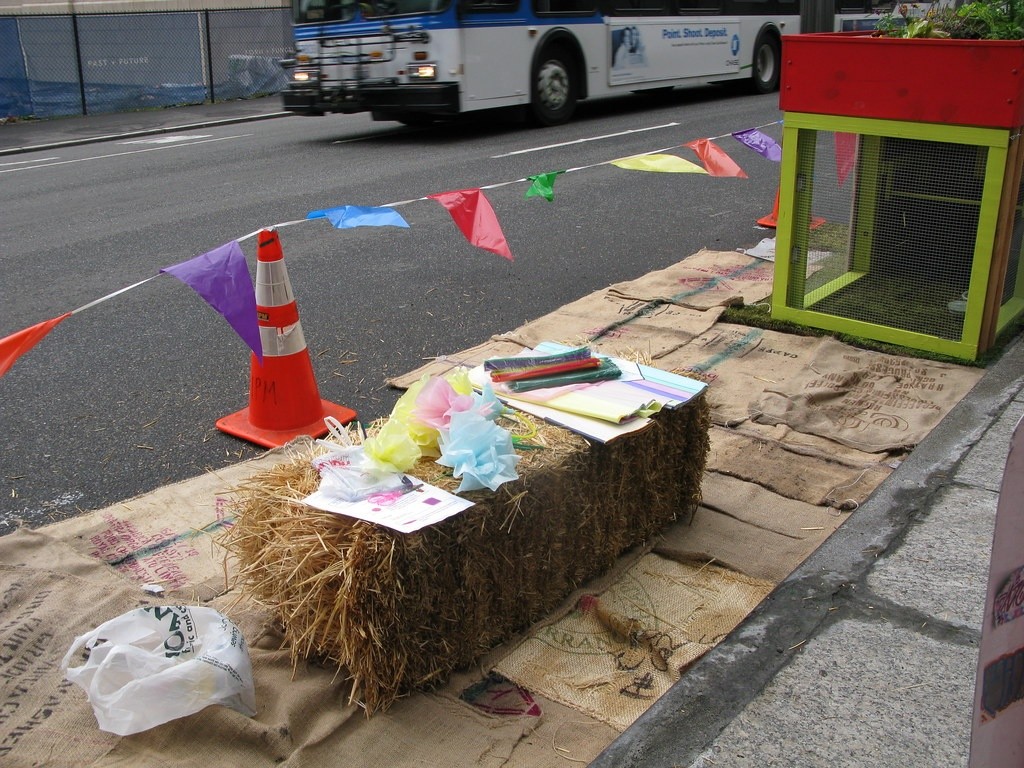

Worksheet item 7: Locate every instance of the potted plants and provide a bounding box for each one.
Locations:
[779,0,1024,128]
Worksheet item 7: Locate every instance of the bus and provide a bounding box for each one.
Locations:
[279,0,965,141]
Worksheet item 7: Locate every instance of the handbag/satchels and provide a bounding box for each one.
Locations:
[60,606,257,736]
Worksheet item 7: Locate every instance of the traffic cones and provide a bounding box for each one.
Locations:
[215,228,356,449]
[758,181,826,232]
